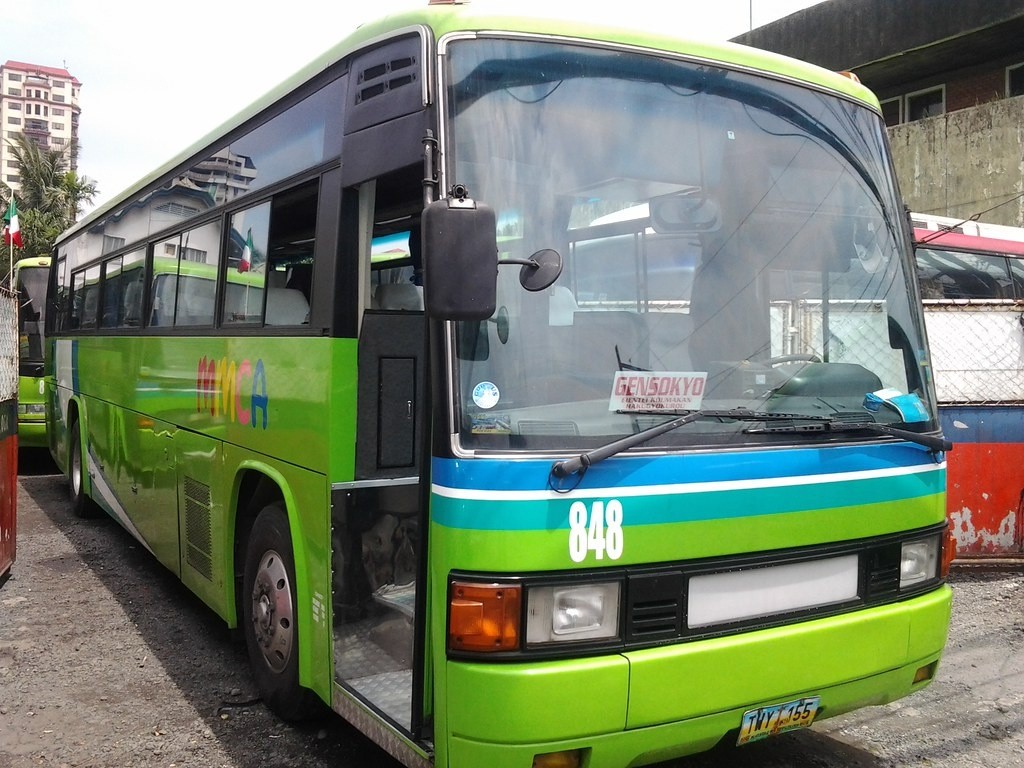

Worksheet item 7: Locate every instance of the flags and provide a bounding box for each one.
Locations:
[237,228,253,273]
[3,200,23,250]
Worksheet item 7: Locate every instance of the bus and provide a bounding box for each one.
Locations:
[565,192,1024,412]
[363,232,525,304]
[42,7,958,768]
[10,254,56,452]
[68,254,264,330]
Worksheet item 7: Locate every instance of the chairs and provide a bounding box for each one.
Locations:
[691,232,766,400]
[81,266,579,327]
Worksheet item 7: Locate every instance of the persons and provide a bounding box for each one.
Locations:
[506,242,578,325]
